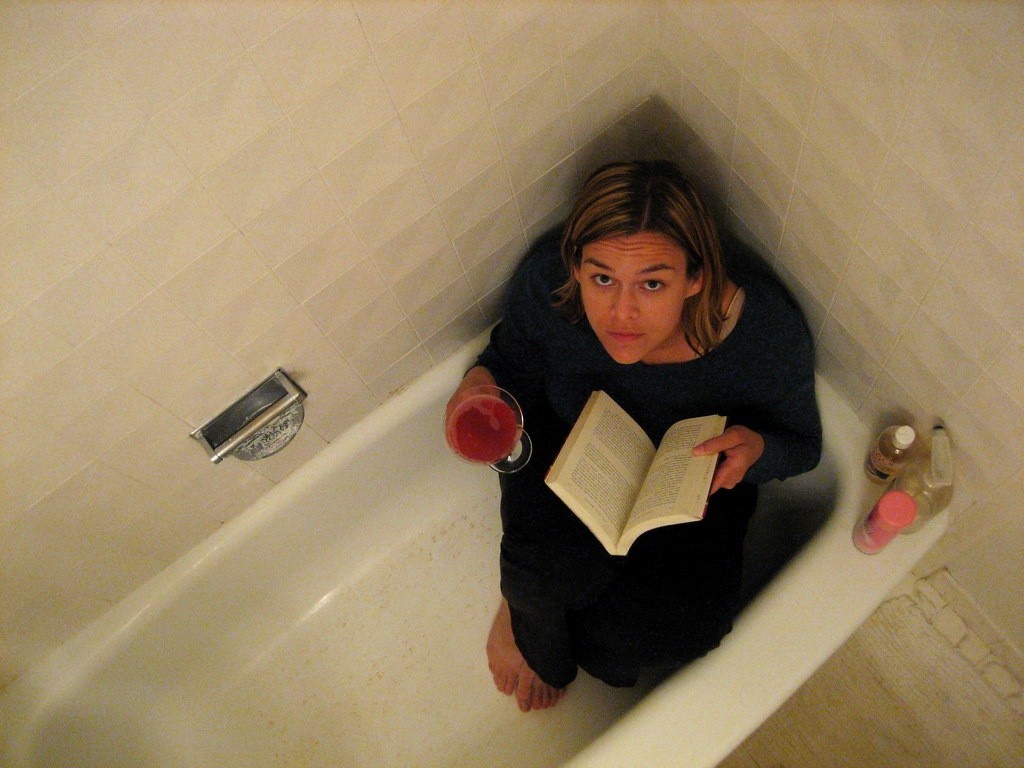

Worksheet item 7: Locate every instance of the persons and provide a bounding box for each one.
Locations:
[443,153,828,713]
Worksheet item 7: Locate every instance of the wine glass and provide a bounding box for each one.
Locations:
[443,385,532,473]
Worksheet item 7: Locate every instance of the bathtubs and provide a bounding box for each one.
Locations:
[3,320,965,768]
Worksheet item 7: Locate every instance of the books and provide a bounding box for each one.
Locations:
[544,388,728,557]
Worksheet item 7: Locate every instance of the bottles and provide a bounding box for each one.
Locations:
[864,425,916,486]
[852,490,917,556]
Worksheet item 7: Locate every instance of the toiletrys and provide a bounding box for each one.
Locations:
[852,490,918,556]
[865,422,920,485]
[881,425,953,535]
